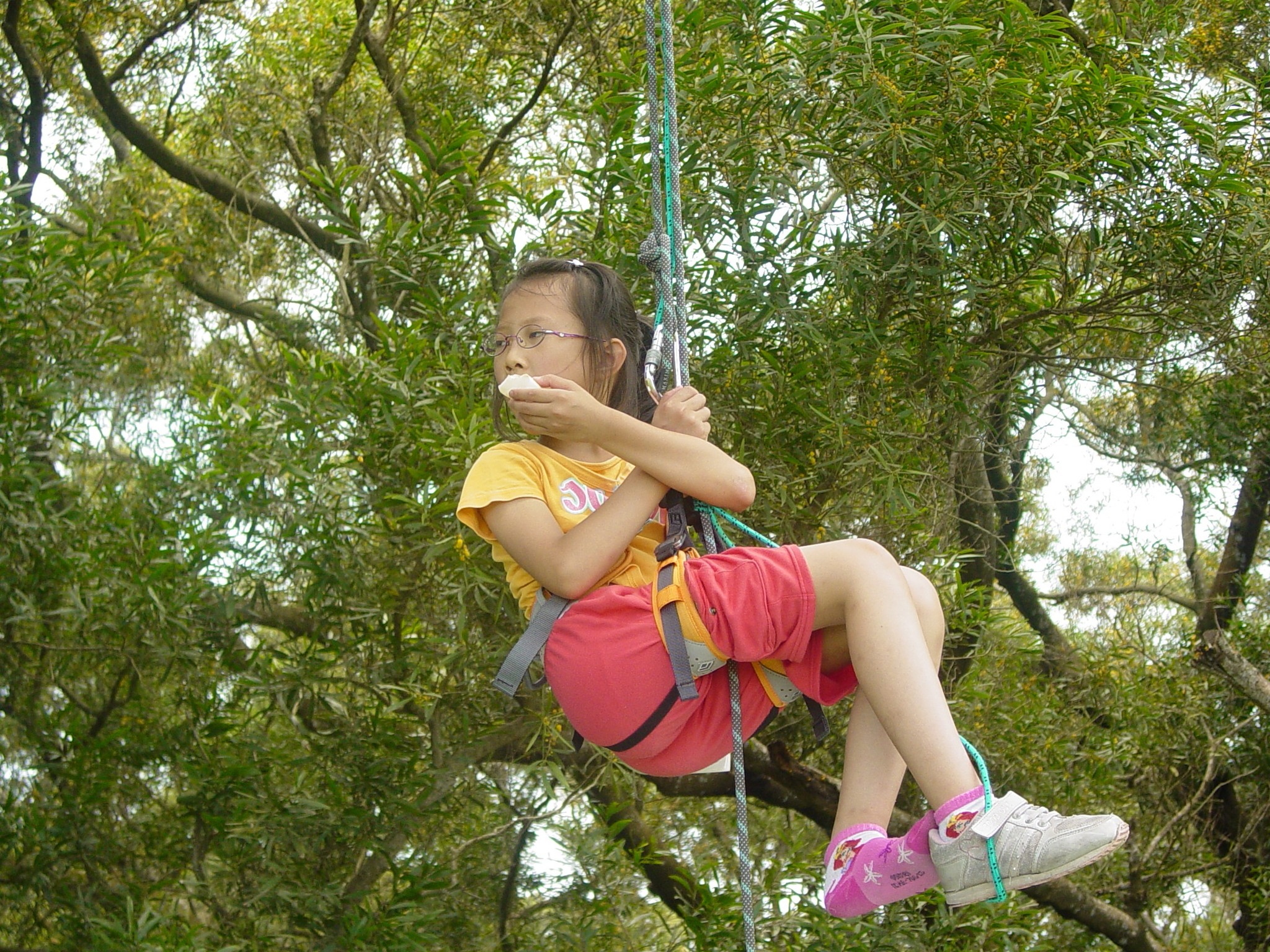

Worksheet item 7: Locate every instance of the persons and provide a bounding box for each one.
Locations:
[453,261,1130,921]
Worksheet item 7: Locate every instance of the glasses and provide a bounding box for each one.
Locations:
[480,324,607,357]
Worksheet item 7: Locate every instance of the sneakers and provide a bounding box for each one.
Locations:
[928,791,1130,907]
